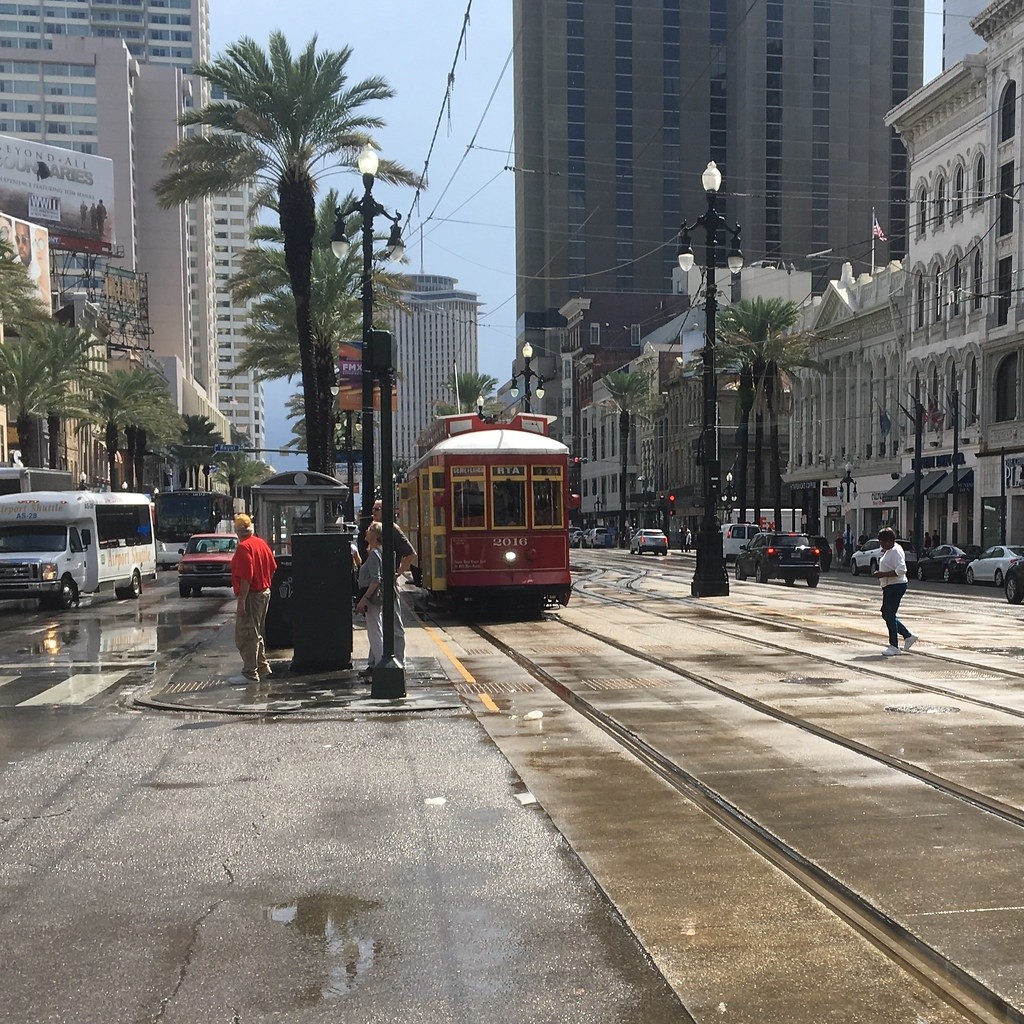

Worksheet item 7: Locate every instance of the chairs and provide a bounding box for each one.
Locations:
[200,544,207,552]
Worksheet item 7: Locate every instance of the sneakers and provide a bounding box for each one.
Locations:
[903,635,918,651]
[882,645,901,655]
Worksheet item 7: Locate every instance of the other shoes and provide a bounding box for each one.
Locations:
[228,675,260,685]
[364,677,372,684]
[260,672,272,678]
[358,668,376,676]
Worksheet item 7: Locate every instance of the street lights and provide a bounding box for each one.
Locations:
[676,158,746,598]
[509,341,546,413]
[330,373,363,526]
[720,471,739,524]
[326,141,408,605]
[837,462,859,567]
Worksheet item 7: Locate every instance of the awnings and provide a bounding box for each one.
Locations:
[926,469,974,499]
[882,473,924,501]
[904,471,948,502]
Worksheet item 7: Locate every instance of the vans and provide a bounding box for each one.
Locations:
[720,522,762,565]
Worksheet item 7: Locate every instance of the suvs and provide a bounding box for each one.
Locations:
[735,530,822,587]
[850,537,919,578]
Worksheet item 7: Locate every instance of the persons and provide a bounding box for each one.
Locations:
[229,514,277,684]
[873,527,919,655]
[931,530,940,548]
[80,201,88,224]
[679,525,692,553]
[351,544,362,567]
[924,532,931,549]
[0,215,52,316]
[834,527,869,563]
[907,529,912,542]
[355,522,383,677]
[895,530,902,539]
[371,497,419,667]
[90,199,107,237]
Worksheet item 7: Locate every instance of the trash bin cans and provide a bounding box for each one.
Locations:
[289,530,356,676]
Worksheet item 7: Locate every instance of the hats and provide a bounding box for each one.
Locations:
[235,513,251,528]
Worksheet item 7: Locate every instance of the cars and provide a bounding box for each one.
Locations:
[630,528,668,556]
[568,526,609,549]
[916,543,987,584]
[965,545,1024,588]
[1004,559,1024,605]
[177,533,240,598]
[811,535,833,573]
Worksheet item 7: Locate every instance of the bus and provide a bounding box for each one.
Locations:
[395,411,573,611]
[151,486,245,571]
[0,489,158,611]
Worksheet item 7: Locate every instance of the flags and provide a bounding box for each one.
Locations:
[879,409,891,437]
[874,217,887,241]
[920,404,929,427]
[927,395,945,432]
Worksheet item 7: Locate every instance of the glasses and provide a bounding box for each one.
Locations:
[372,507,380,511]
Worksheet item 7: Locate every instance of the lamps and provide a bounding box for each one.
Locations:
[930,442,939,447]
[960,438,970,444]
[890,473,899,480]
[905,448,913,453]
